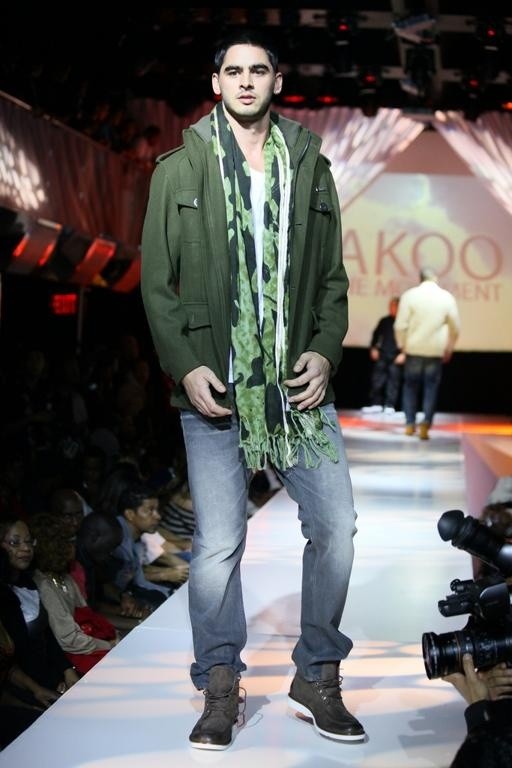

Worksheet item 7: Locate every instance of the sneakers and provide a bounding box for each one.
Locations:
[287,664,366,741]
[363,405,395,415]
[189,667,239,750]
[407,423,429,439]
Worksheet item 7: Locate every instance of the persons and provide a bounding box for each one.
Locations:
[1,332,286,750]
[140,33,370,748]
[392,266,462,442]
[442,499,511,767]
[367,296,402,416]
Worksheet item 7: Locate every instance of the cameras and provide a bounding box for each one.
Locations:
[421,510,512,678]
